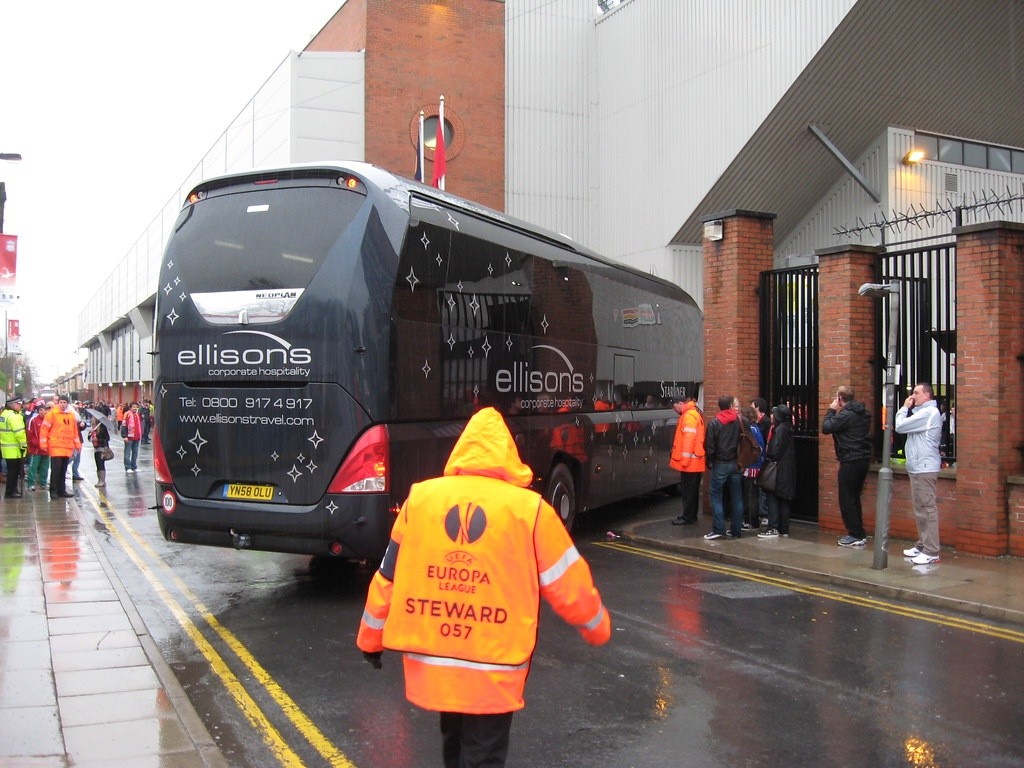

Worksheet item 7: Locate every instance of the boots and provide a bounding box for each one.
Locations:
[96,470,106,487]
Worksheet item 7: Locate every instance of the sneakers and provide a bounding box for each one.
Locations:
[741,523,757,531]
[911,551,940,565]
[838,536,868,546]
[704,531,722,540]
[903,546,922,557]
[725,530,738,539]
[757,528,779,538]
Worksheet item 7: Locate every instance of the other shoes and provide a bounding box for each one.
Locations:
[671,519,690,525]
[58,491,75,497]
[73,475,84,480]
[126,468,141,474]
[6,492,22,499]
[678,516,685,520]
[52,490,57,498]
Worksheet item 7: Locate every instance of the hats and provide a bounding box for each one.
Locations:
[773,404,792,422]
[7,395,20,404]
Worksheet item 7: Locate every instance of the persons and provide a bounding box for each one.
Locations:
[475,388,666,464]
[68,401,154,488]
[895,383,943,565]
[356,407,611,768]
[0,393,82,499]
[669,395,706,526]
[881,398,955,469]
[822,385,872,547]
[704,395,797,539]
[786,400,807,430]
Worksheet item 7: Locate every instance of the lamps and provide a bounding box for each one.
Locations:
[902,149,924,165]
[704,219,724,241]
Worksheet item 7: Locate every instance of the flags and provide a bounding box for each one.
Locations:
[431,110,448,190]
[414,119,425,182]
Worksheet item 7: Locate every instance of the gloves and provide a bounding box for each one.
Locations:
[364,649,382,669]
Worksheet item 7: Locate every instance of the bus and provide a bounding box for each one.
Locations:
[149,161,705,571]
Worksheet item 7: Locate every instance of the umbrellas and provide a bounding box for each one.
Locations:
[85,409,118,437]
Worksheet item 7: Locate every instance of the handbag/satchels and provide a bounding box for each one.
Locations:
[102,442,114,461]
[753,459,784,491]
[734,415,759,468]
